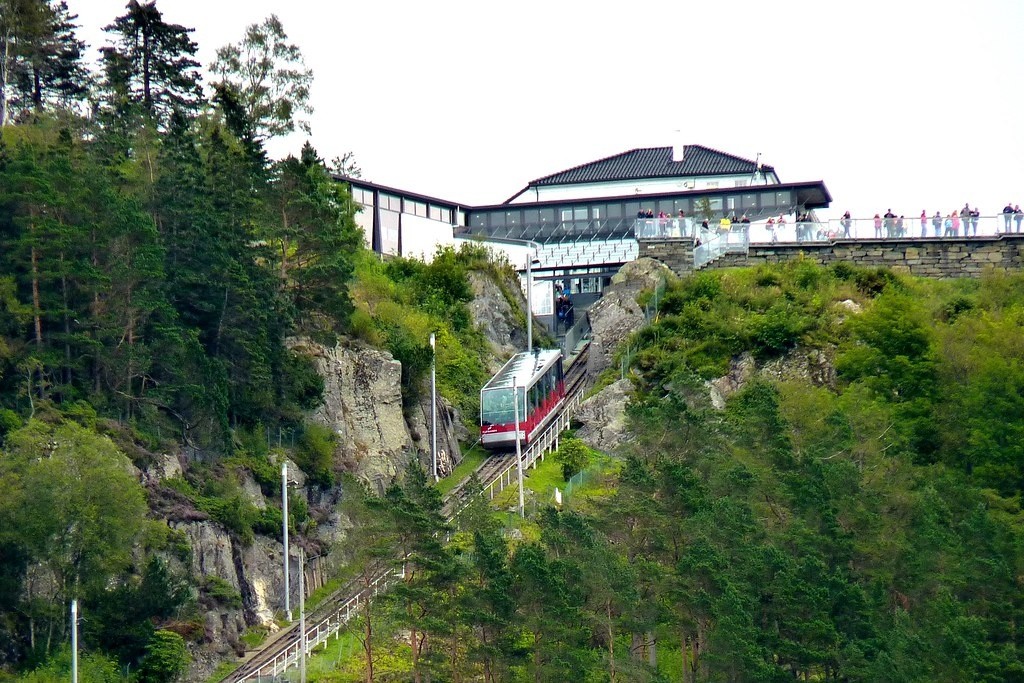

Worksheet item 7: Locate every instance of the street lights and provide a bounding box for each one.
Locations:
[282,463,299,623]
[72,599,88,682]
[527,253,540,351]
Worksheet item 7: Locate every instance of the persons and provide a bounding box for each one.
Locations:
[716,214,750,243]
[764,212,788,242]
[555,284,573,321]
[699,218,710,246]
[919,209,928,237]
[489,375,548,416]
[638,208,688,238]
[794,211,853,241]
[694,237,702,247]
[933,202,980,236]
[1002,200,1024,232]
[873,208,907,238]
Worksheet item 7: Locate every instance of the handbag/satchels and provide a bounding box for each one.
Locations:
[841,217,845,226]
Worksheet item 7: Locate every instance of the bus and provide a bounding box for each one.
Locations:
[480,348,566,449]
